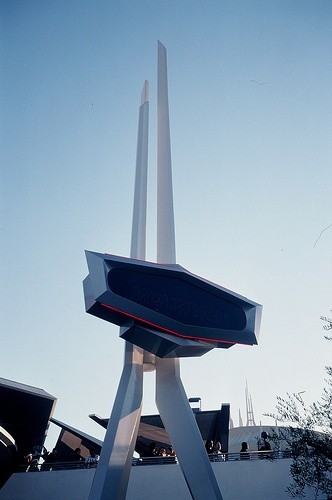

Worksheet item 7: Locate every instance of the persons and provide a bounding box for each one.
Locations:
[25,447,101,473]
[146,443,178,464]
[239,442,252,460]
[203,440,227,462]
[258,431,272,458]
